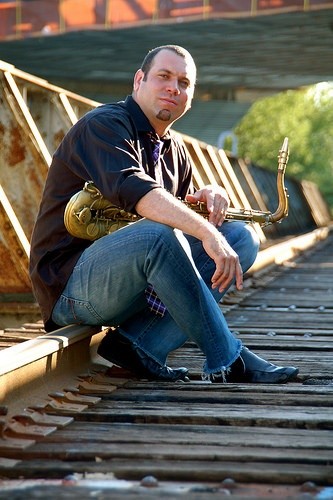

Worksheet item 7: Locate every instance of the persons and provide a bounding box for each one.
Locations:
[29,45,300,384]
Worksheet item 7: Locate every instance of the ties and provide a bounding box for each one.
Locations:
[147,131,164,190]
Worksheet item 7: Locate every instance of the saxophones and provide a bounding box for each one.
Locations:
[63,134,290,241]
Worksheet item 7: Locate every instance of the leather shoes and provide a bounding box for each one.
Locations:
[209,346,299,384]
[96,328,189,382]
